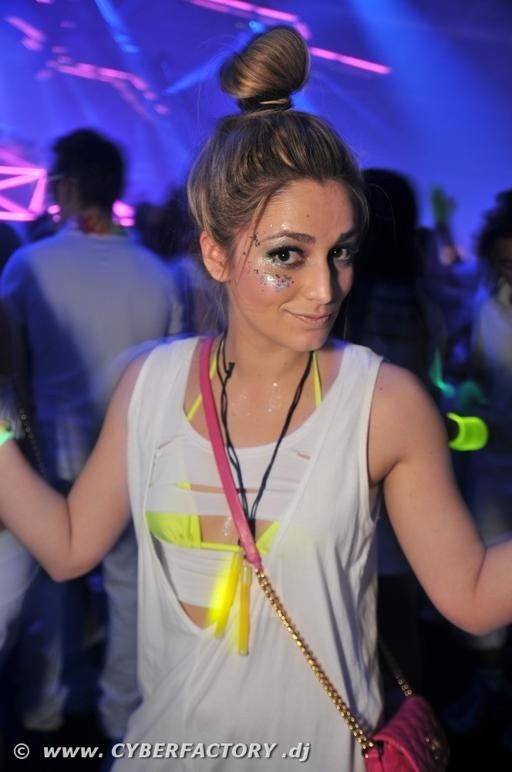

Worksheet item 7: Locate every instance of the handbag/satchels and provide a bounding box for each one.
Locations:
[362,695,450,772]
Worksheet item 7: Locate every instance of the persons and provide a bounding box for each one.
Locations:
[0,23,511,770]
[2,121,511,771]
